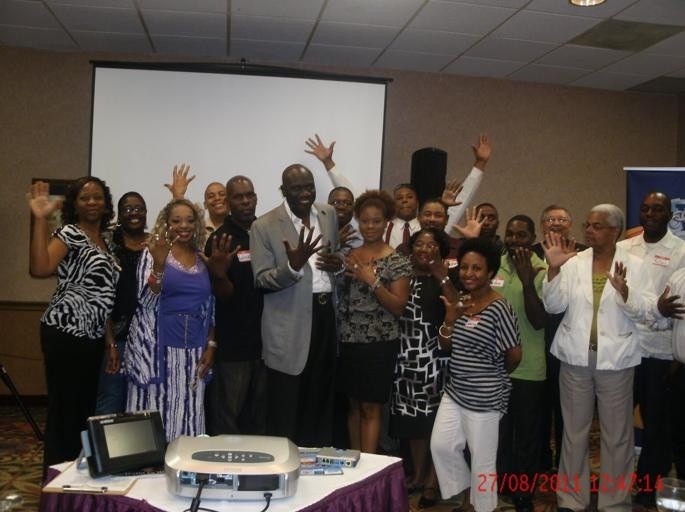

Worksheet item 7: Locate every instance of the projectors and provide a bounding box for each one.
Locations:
[162,435,302,502]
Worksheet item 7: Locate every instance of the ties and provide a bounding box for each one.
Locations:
[402,222,411,256]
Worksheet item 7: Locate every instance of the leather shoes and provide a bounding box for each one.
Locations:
[406,481,418,495]
[419,486,438,509]
[515,495,534,512]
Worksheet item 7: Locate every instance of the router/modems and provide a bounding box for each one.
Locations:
[316,445,362,468]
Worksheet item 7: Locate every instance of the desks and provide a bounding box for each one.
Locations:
[41,442,409,512]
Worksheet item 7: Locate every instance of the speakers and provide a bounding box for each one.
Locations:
[410,146,447,203]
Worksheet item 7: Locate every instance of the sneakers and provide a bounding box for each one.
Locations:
[559,507,574,511]
[452,506,467,511]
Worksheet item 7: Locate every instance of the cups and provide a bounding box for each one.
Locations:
[655,476,685,512]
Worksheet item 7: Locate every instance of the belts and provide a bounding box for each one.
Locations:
[589,342,597,351]
[312,292,334,306]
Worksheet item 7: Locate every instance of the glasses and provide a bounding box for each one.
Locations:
[543,216,571,225]
[582,221,617,230]
[120,206,146,214]
